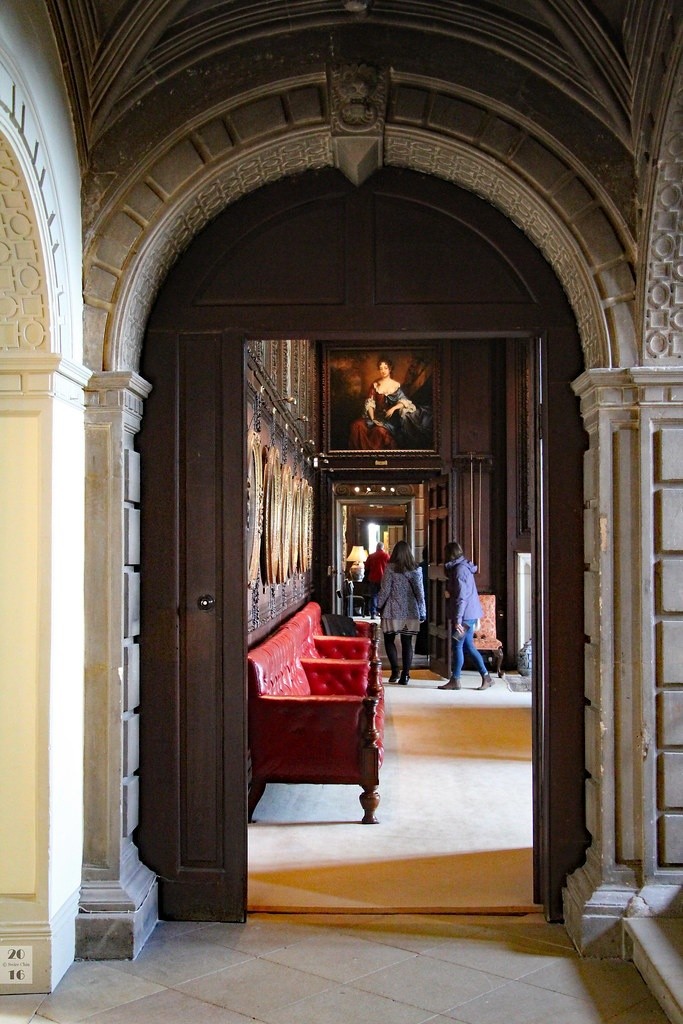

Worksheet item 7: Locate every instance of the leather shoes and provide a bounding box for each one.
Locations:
[388,665,400,682]
[398,672,409,685]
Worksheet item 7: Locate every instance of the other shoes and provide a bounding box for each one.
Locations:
[437,676,461,690]
[371,611,375,619]
[477,672,494,690]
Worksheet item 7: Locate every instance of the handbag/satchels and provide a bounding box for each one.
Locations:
[419,604,425,621]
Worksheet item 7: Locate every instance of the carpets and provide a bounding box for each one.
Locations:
[504,676,532,692]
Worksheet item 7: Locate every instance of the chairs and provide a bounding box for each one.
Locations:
[472,595,505,678]
[343,578,365,617]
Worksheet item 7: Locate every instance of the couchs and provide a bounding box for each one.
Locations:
[247,601,384,826]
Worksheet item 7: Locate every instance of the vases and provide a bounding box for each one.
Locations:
[518,644,532,676]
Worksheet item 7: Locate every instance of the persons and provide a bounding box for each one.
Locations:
[364,542,390,619]
[437,542,495,690]
[376,541,426,686]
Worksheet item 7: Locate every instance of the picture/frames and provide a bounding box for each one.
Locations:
[320,341,442,462]
[247,429,313,590]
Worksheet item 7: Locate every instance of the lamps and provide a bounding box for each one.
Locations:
[248,396,334,481]
[345,545,368,582]
